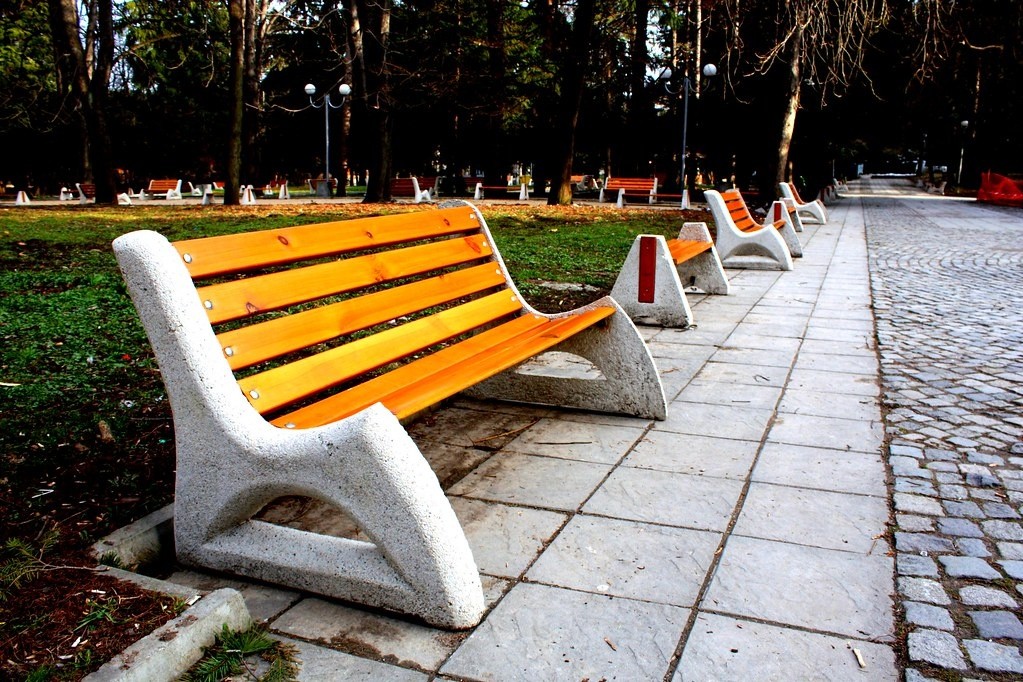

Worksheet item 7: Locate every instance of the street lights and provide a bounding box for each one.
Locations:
[305,83,352,183]
[658,62,718,194]
[956,120,971,188]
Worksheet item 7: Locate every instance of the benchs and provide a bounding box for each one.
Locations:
[616,188,691,211]
[703,177,848,272]
[472,183,529,201]
[916,178,948,195]
[112,199,669,631]
[607,221,727,327]
[599,177,659,203]
[0,179,439,206]
